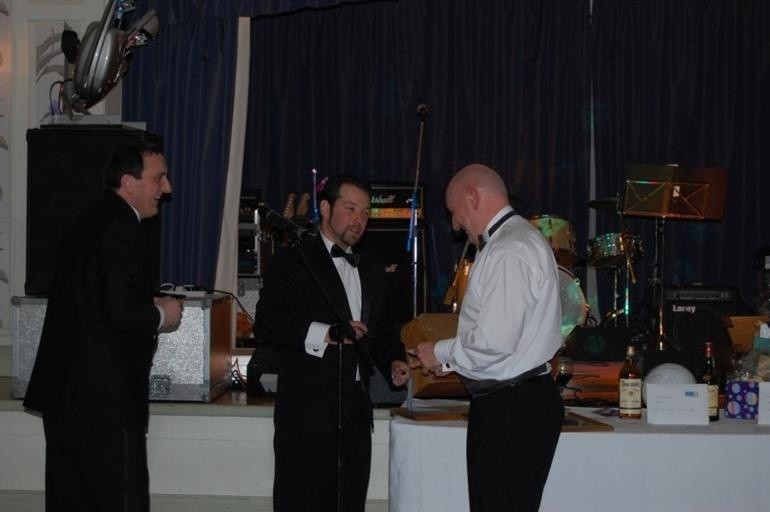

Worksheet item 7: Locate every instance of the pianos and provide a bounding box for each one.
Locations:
[664,284,733,304]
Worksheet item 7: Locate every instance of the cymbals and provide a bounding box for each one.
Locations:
[589,199,624,214]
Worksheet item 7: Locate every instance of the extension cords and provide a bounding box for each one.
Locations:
[158,283,209,298]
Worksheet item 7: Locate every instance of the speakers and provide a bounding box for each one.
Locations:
[24,122,146,298]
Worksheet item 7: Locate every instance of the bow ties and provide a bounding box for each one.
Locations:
[332,243,359,269]
[465,235,486,263]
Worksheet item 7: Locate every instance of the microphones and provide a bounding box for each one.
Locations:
[258,203,309,242]
[417,103,429,121]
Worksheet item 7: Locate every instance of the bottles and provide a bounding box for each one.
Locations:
[699,344,719,421]
[619,345,642,419]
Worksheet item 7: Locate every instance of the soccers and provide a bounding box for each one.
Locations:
[641,362,696,410]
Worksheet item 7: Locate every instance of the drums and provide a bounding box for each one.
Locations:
[527,211,589,339]
[584,231,647,272]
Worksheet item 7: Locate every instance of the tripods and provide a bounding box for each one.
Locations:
[646,227,684,348]
[606,216,645,326]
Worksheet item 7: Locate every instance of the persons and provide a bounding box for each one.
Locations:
[23,141,184,512]
[409,163,564,512]
[252,172,409,512]
